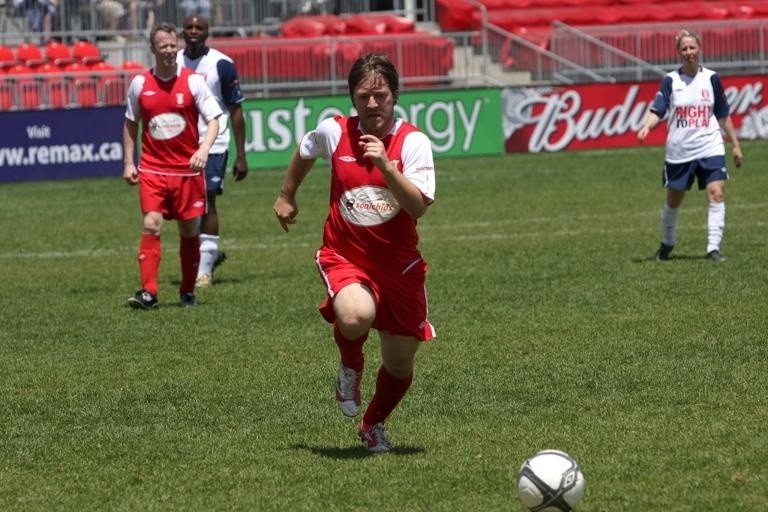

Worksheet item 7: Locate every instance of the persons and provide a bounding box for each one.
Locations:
[637,30,743,262]
[175,15,248,288]
[0,0,224,45]
[122,23,224,310]
[273,51,438,454]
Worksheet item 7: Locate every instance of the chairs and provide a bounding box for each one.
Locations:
[1,1,768,117]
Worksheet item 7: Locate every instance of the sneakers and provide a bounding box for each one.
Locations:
[127,288,161,312]
[356,420,392,454]
[335,361,362,417]
[194,275,216,290]
[705,248,728,264]
[178,294,197,309]
[655,242,676,260]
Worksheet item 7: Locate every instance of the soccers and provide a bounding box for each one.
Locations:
[516,449,585,511]
